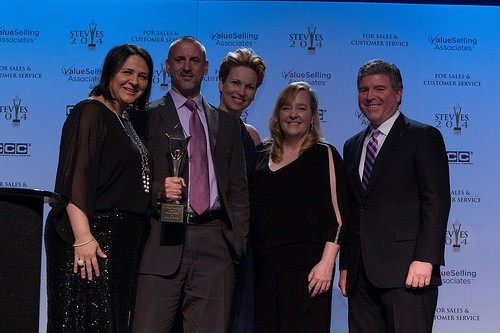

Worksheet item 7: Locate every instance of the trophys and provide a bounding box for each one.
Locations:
[158,133,193,224]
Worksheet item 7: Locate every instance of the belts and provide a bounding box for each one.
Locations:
[184,210,225,225]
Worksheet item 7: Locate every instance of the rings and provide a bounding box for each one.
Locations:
[77,260,85,267]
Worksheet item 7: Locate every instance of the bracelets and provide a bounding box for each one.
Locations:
[72,238,94,247]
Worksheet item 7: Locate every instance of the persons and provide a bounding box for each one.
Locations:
[129,35,248,333]
[216,48,266,333]
[248,81,344,333]
[337,59,450,333]
[43,44,151,333]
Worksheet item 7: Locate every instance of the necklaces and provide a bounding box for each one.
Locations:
[109,102,150,193]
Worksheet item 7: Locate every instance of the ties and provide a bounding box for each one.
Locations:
[183,100,210,215]
[361,129,381,192]
